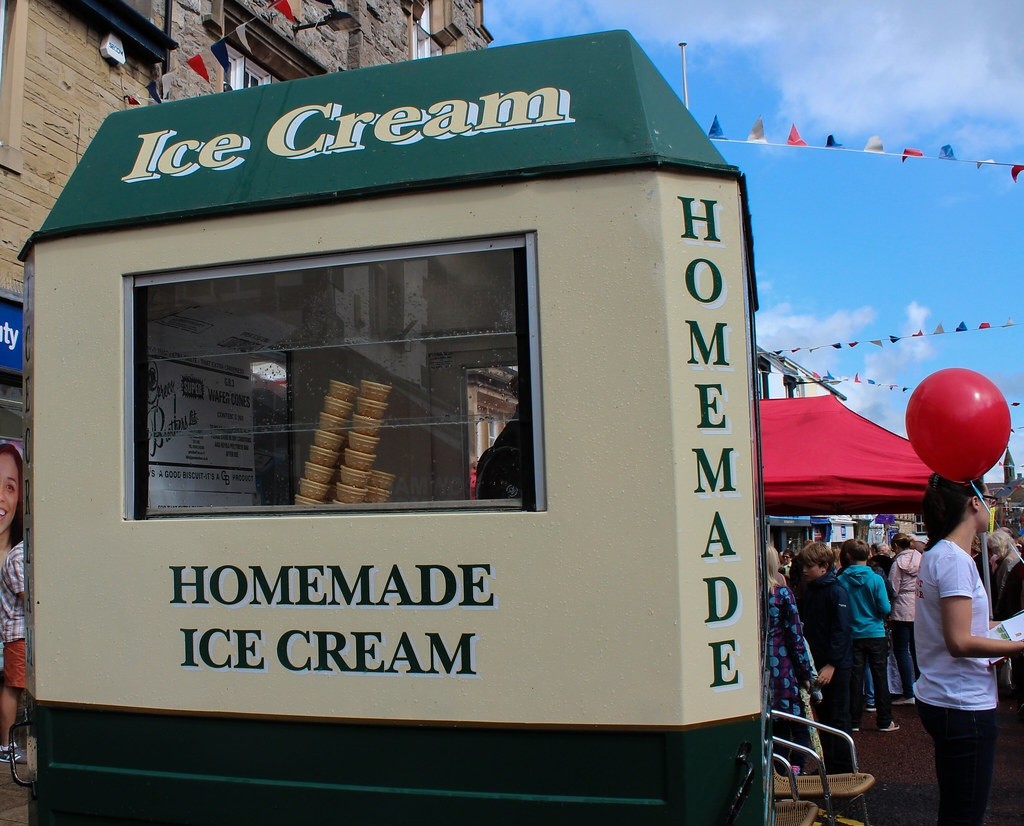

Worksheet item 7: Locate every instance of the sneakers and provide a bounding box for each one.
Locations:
[0,743,28,764]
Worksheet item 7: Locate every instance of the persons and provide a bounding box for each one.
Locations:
[766,525,1024,775]
[0,540,27,765]
[0,441,23,571]
[911,473,1024,826]
[476,371,521,499]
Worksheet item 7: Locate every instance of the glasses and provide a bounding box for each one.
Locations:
[890,546,894,552]
[973,493,999,508]
[782,555,791,558]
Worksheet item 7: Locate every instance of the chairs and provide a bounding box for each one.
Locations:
[770,709,876,826]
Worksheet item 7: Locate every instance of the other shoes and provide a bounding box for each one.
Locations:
[878,720,900,732]
[890,696,916,705]
[1016,703,1024,713]
[791,765,802,776]
[864,703,877,712]
[850,722,861,732]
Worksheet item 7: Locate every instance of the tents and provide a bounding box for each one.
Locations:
[759,392,934,516]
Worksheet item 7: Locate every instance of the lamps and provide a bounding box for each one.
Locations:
[293,8,362,31]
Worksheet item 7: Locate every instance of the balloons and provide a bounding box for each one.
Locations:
[905,368,1011,484]
